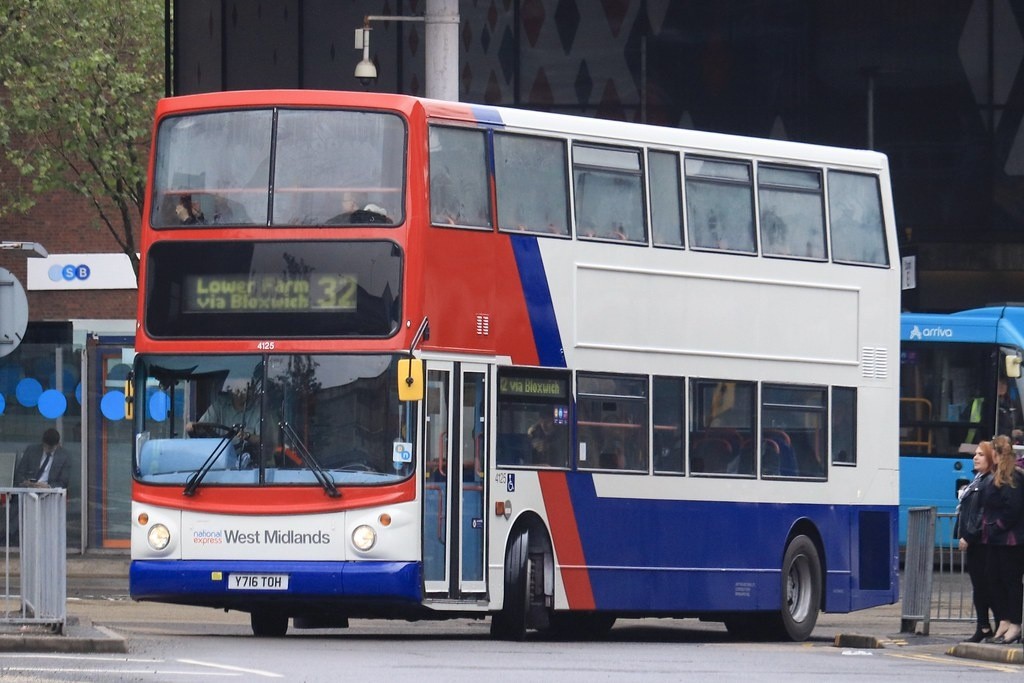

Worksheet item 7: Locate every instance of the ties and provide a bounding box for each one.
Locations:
[32,452,52,483]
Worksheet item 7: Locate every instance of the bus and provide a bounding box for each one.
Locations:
[125,88,902,640]
[894,304,1024,552]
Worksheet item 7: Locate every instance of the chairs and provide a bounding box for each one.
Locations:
[600,453,624,468]
[0,453,71,511]
[437,213,629,240]
[692,427,798,476]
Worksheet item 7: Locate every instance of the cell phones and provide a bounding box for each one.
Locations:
[30,479,38,483]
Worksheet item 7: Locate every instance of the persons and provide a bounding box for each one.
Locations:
[527,422,548,465]
[186,373,280,468]
[537,404,568,468]
[339,188,387,216]
[12,429,73,499]
[952,434,1024,643]
[175,170,253,225]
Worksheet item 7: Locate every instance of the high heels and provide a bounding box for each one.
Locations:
[986,628,1021,644]
[964,628,993,642]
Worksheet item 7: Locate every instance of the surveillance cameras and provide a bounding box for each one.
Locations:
[354,62,377,87]
